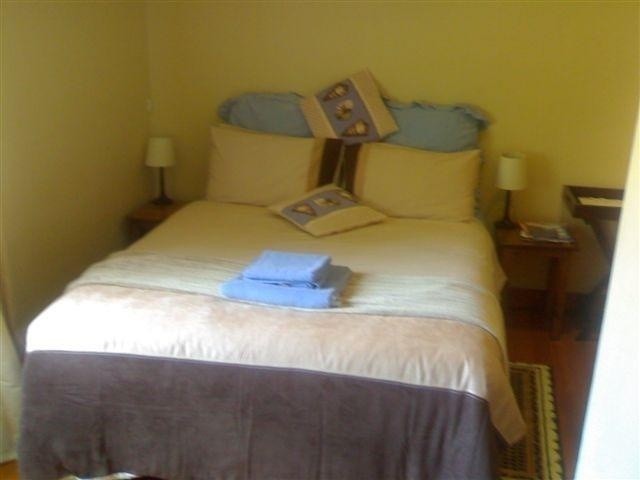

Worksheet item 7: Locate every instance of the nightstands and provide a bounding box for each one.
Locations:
[486,218,577,339]
[123,200,195,249]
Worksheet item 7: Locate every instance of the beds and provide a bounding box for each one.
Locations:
[15,69,528,480]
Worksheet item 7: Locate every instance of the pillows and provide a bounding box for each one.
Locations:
[298,67,399,145]
[215,90,315,138]
[203,121,346,207]
[335,141,484,222]
[379,95,490,153]
[267,182,387,238]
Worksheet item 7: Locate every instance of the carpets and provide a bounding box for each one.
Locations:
[492,361,566,480]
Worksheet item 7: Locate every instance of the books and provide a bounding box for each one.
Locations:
[519,221,574,243]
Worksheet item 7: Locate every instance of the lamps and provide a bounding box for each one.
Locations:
[492,151,527,232]
[143,137,179,206]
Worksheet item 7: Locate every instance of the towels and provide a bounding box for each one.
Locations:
[221,264,353,312]
[244,248,333,288]
